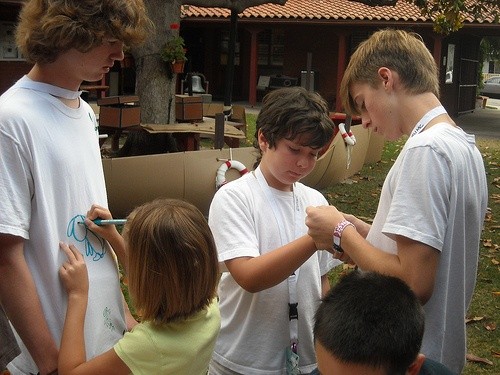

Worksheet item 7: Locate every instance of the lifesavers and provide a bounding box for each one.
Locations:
[215,160,248,189]
[338,123,357,147]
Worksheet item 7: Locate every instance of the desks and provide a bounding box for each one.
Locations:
[142,118,246,152]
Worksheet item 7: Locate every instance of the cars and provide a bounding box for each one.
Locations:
[479,76,500,95]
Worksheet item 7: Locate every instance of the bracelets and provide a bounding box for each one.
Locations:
[332,220,356,252]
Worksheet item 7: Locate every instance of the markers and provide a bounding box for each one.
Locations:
[77,220,127,225]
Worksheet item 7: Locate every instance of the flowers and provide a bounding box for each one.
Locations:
[154,21,188,67]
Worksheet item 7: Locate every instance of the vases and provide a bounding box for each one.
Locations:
[172,59,186,74]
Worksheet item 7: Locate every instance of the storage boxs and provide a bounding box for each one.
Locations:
[96,93,143,128]
[175,93,204,122]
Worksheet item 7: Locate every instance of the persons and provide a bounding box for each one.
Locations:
[311,271,457,375]
[0,0,157,375]
[58,198,221,375]
[208,87,355,375]
[305,30,487,375]
